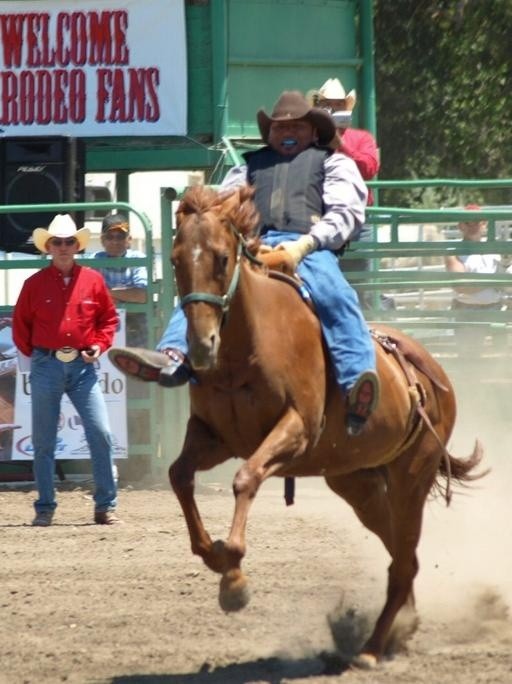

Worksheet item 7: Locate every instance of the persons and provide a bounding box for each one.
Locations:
[305,78,381,301]
[13,212,125,530]
[90,212,148,403]
[109,92,382,435]
[441,201,511,377]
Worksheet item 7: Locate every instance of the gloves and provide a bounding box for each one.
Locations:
[274,235,317,267]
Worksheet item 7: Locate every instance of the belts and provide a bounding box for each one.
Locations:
[35,346,89,363]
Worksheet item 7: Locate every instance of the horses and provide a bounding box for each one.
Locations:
[168,174,493,675]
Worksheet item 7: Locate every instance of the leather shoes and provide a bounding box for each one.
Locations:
[344,371,379,438]
[107,347,191,386]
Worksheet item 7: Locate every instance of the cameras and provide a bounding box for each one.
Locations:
[323,108,352,127]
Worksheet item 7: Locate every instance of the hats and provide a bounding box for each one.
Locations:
[32,214,90,253]
[103,214,129,232]
[257,91,334,146]
[305,78,356,111]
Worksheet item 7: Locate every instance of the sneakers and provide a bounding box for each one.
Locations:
[94,512,121,524]
[32,514,52,525]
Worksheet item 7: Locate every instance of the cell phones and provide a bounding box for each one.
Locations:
[86,350,96,356]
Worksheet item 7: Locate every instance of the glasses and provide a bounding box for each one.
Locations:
[50,239,75,245]
[106,233,126,240]
